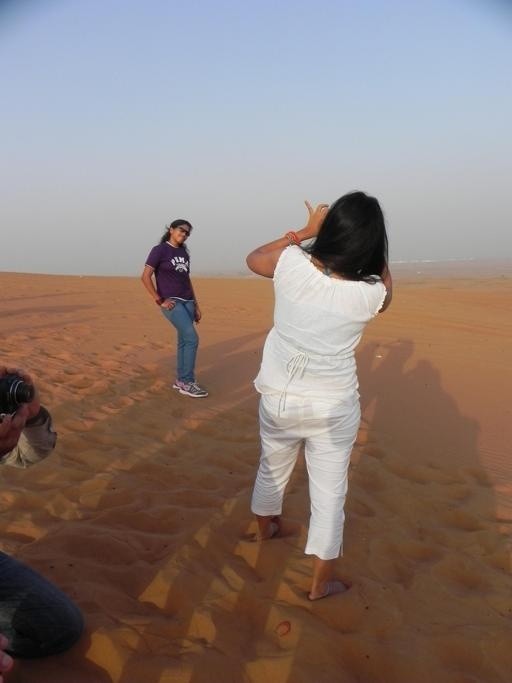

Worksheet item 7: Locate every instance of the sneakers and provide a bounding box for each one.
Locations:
[172,379,208,397]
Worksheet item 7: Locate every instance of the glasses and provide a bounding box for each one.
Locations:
[175,226,190,236]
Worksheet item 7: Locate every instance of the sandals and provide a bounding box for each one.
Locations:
[259,517,281,539]
[307,579,348,601]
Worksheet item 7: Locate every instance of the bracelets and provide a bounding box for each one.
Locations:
[155,296,164,305]
[286,230,302,246]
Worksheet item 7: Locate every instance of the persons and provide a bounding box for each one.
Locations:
[243,188,391,602]
[142,218,210,398]
[0,365,86,683]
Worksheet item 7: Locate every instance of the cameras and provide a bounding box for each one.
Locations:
[0,371,35,421]
